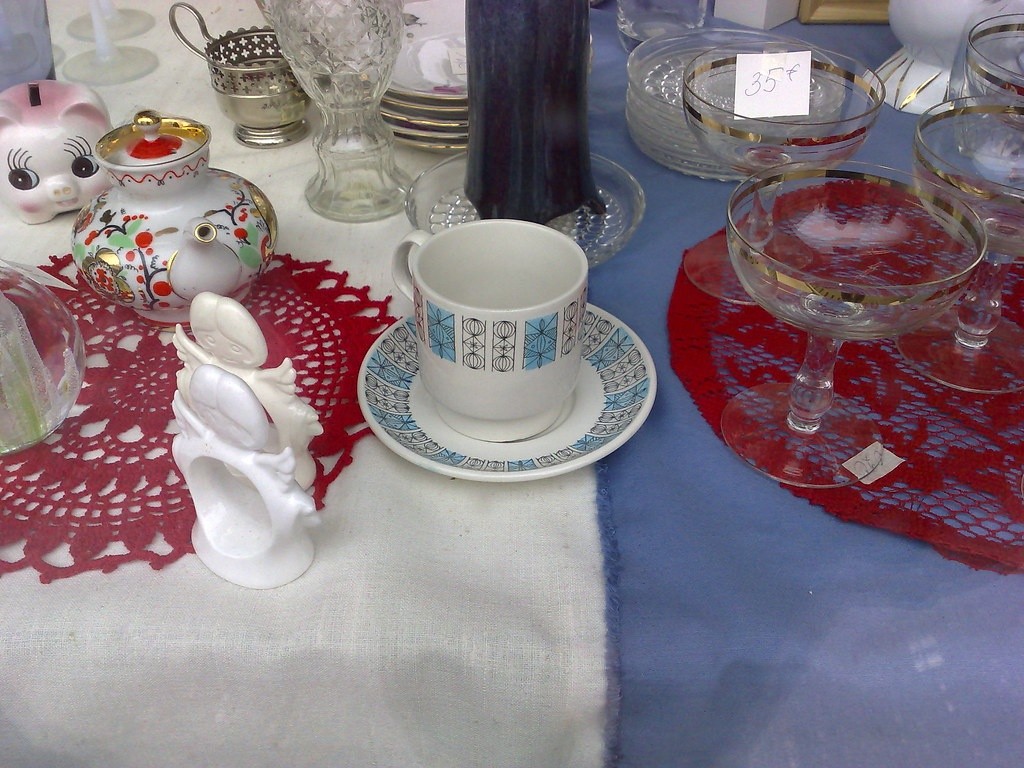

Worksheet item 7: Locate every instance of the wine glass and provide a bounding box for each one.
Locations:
[894,91,1024,394]
[681,40,885,304]
[721,159,989,491]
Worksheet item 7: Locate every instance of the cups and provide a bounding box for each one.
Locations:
[391,218,589,420]
[965,12,1024,133]
[617,0,708,58]
[256,0,413,222]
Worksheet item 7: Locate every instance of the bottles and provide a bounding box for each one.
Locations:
[463,0,607,220]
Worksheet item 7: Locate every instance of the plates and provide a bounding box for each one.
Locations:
[401,155,645,268]
[357,300,658,483]
[366,0,472,153]
[624,27,847,181]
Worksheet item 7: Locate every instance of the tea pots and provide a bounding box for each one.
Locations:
[69,110,278,323]
